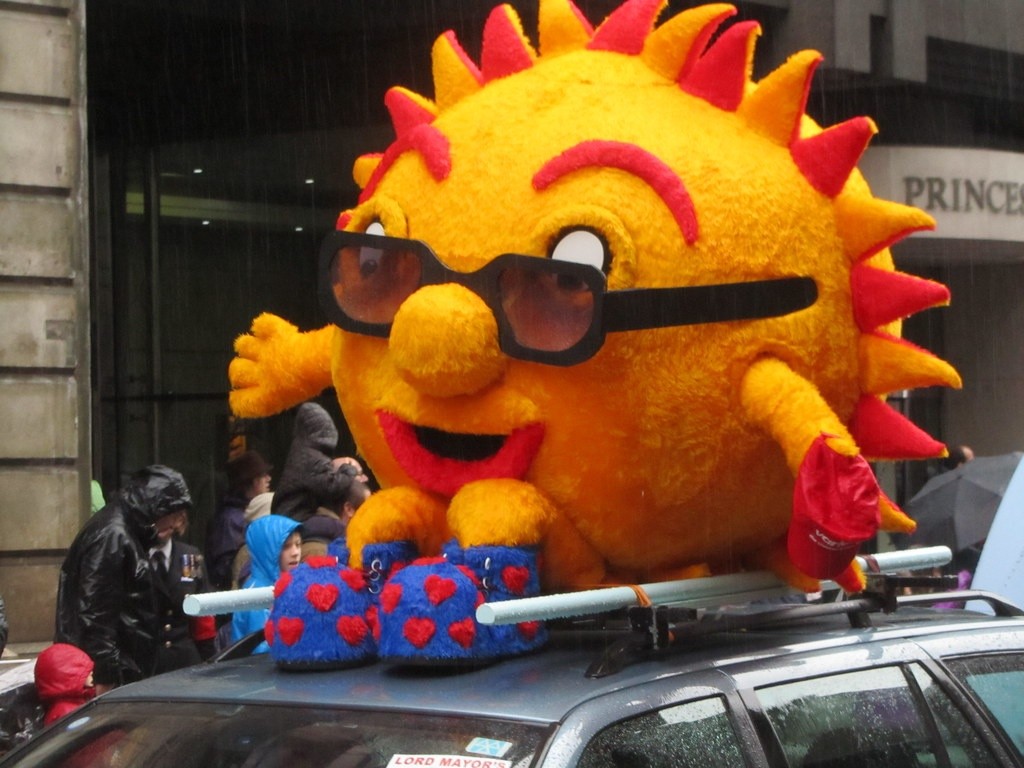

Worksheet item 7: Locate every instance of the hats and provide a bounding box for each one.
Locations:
[223,449,275,493]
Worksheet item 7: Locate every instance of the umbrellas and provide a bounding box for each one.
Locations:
[886,451,1023,555]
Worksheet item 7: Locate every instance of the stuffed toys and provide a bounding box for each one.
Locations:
[231,0,964,665]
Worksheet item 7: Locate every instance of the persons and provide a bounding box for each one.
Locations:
[145,535,217,680]
[901,543,943,599]
[53,463,194,696]
[204,401,371,655]
[946,445,973,470]
[33,643,130,768]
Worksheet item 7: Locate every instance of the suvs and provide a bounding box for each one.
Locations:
[2,586,1024,768]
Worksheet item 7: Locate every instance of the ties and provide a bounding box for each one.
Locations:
[153,552,168,582]
